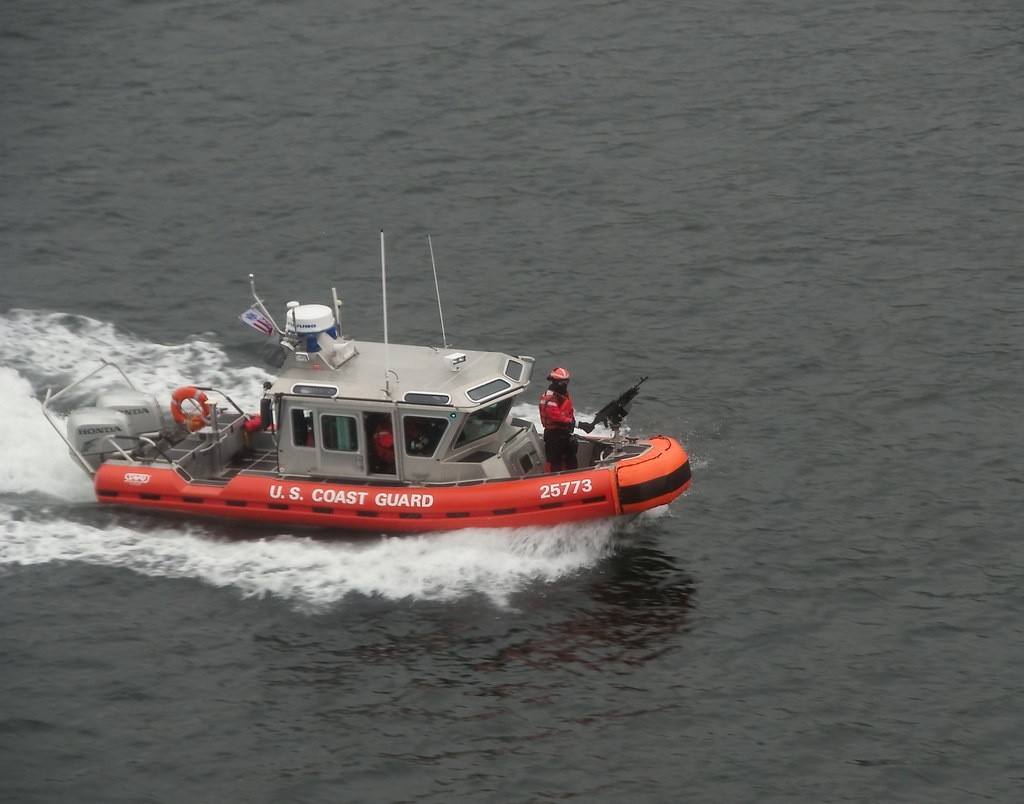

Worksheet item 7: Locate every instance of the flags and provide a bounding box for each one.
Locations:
[239,308,274,337]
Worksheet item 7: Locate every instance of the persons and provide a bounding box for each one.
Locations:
[538,366,594,476]
[372,412,429,474]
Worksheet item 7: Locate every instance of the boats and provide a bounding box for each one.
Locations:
[43,232,694,532]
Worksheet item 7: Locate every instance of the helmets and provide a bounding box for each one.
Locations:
[549,368,570,381]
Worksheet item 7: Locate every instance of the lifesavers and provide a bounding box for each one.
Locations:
[170,388,211,428]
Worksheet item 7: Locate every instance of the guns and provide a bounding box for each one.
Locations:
[591,375,649,428]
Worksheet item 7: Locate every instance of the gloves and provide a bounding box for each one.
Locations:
[579,421,595,434]
